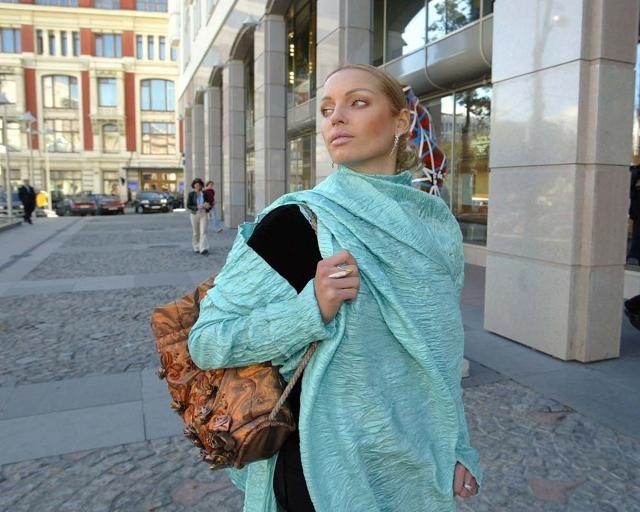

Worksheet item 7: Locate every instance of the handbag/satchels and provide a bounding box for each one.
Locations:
[151,275,298,471]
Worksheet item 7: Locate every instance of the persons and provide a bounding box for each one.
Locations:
[186,62,484,512]
[127,187,132,202]
[18,178,36,224]
[204,181,223,233]
[624,163,640,333]
[187,177,211,255]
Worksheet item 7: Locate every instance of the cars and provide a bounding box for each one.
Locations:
[54,193,125,217]
[132,190,170,212]
[162,190,182,207]
[0,191,26,218]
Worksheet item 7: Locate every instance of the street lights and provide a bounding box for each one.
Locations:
[0,91,18,219]
[19,110,69,219]
[525,12,572,148]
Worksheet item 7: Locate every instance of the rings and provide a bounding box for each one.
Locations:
[463,484,475,494]
[327,266,351,280]
